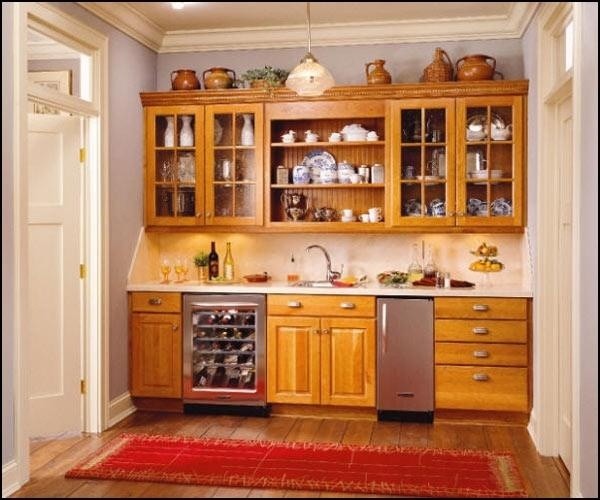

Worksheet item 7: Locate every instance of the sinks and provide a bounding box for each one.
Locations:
[287,279,334,288]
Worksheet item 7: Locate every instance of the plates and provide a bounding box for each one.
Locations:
[466,111,505,140]
[466,169,503,179]
[415,175,443,180]
[302,151,337,181]
[244,274,272,281]
[213,118,223,146]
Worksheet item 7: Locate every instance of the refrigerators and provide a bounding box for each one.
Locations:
[178,292,268,412]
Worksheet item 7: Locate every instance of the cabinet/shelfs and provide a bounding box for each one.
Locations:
[384,72,532,239]
[433,278,535,430]
[262,74,397,239]
[134,81,265,241]
[124,287,185,421]
[262,285,379,423]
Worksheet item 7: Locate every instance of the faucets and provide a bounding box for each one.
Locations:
[305,244,339,276]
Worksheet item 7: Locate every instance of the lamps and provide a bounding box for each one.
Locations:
[281,0,339,101]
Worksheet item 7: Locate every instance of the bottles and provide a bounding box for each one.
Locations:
[279,192,312,222]
[209,241,218,279]
[292,163,310,184]
[241,114,254,146]
[336,161,355,185]
[223,241,236,280]
[424,243,437,282]
[358,165,369,183]
[365,59,392,85]
[165,116,174,146]
[202,67,236,89]
[437,271,450,288]
[287,252,300,283]
[409,240,421,283]
[315,208,338,222]
[191,307,256,389]
[371,163,385,183]
[455,54,496,81]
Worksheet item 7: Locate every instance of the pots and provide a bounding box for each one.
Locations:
[217,158,241,182]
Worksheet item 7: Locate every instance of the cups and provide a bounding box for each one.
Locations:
[430,130,442,142]
[405,197,512,216]
[348,174,363,184]
[337,206,382,223]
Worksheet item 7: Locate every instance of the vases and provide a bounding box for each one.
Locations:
[239,112,255,147]
[163,116,176,148]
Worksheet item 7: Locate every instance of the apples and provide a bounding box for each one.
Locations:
[476,244,497,254]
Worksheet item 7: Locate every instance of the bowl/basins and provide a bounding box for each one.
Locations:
[466,131,485,141]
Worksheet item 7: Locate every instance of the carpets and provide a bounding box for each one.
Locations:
[63,430,534,500]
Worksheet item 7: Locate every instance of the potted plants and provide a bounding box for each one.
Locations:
[193,253,210,281]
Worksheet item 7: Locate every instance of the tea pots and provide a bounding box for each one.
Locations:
[171,70,201,90]
[491,124,511,141]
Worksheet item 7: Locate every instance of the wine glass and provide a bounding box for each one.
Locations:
[162,253,189,284]
[160,161,177,182]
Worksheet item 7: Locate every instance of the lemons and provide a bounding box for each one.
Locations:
[470,260,502,271]
